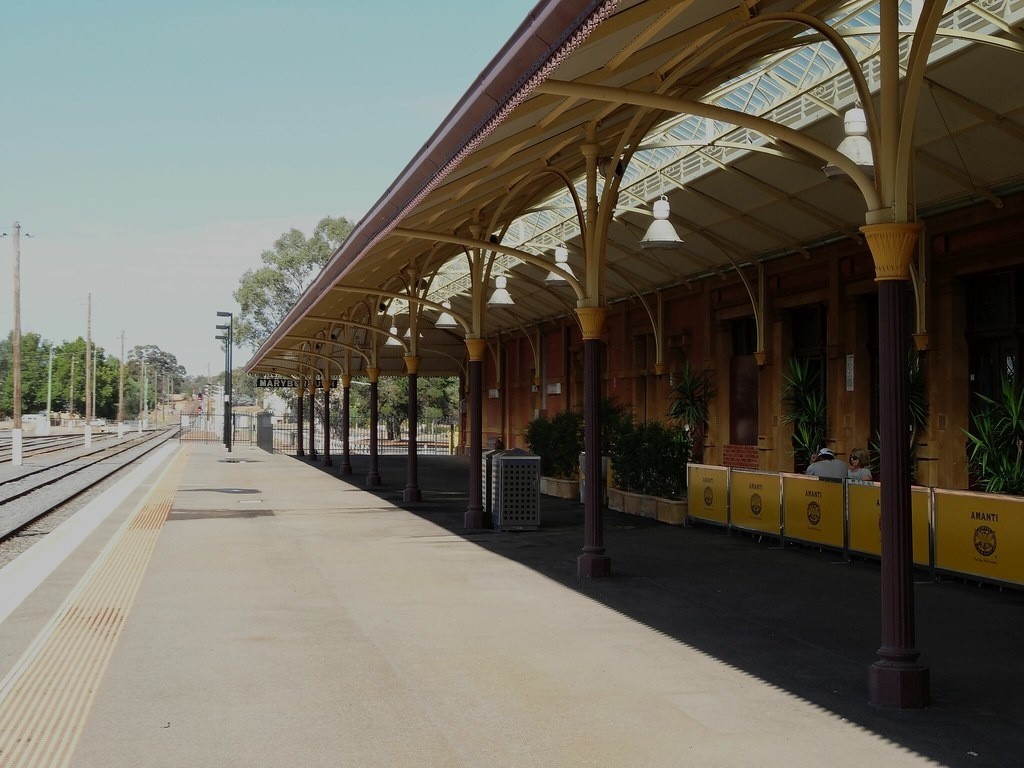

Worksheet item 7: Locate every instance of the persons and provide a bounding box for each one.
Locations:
[847,447,873,481]
[805,447,848,478]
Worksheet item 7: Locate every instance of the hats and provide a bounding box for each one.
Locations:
[818,448,834,457]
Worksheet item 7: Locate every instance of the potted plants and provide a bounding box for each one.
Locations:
[523,392,697,525]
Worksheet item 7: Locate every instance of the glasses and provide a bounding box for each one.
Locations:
[851,455,858,461]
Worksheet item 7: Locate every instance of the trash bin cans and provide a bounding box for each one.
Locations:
[492,448,541,532]
[479,447,502,515]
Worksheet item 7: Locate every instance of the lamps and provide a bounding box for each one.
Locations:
[384,316,404,346]
[544,224,579,286]
[821,82,875,179]
[486,256,516,309]
[434,293,459,329]
[639,170,684,251]
[403,313,423,341]
